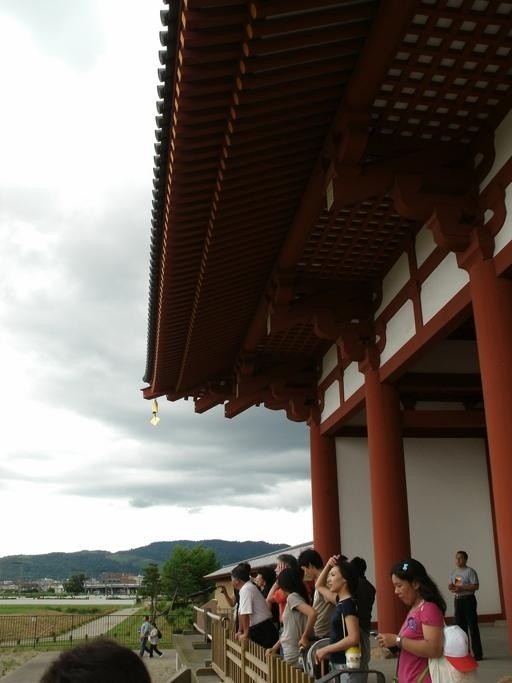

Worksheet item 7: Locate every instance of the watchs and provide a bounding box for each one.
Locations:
[396,636,402,648]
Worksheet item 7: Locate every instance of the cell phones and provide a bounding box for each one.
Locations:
[369,632,378,636]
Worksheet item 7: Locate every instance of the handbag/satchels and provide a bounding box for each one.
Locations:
[420,602,482,683]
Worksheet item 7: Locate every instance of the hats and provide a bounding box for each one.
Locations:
[441,624,480,673]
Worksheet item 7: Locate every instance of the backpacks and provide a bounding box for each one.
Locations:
[158,628,162,639]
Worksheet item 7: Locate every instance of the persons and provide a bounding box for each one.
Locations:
[376,559,447,683]
[148,622,164,657]
[39,638,151,683]
[219,548,375,683]
[449,550,483,660]
[140,616,150,656]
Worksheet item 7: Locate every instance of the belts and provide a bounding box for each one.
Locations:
[455,594,475,599]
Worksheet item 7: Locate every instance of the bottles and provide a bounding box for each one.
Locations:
[345,647,361,670]
